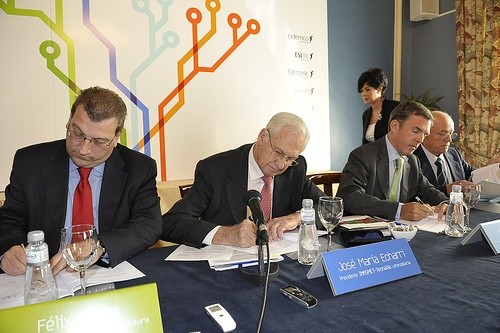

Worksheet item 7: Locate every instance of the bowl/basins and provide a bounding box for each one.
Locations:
[391,226,417,243]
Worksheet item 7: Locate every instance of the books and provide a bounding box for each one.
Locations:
[396,213,446,233]
[0,260,146,309]
[337,215,392,231]
[164,227,334,271]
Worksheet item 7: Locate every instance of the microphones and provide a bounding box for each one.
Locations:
[243,189,269,242]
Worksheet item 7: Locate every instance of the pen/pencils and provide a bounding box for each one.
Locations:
[468,173,474,182]
[248,216,253,222]
[21,243,25,251]
[415,196,424,204]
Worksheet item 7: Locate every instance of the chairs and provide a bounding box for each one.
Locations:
[307,172,343,197]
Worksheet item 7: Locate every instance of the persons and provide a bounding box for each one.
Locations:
[412,111,478,196]
[0,88,163,276]
[335,102,449,221]
[358,68,400,144]
[159,112,340,248]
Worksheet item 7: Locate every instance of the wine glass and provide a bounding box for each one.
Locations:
[461,184,481,231]
[61,224,98,295]
[318,196,343,251]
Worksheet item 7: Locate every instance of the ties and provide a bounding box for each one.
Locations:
[434,158,449,186]
[389,158,404,202]
[71,167,94,244]
[260,176,272,221]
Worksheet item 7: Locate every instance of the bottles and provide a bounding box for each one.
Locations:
[297,199,319,265]
[445,185,465,238]
[23,230,57,304]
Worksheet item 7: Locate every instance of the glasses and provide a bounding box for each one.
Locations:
[431,131,452,136]
[66,116,116,147]
[266,129,298,166]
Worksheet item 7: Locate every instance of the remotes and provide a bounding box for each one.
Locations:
[280,285,318,309]
[205,303,236,332]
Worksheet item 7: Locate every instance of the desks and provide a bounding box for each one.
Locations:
[0,194,500,333]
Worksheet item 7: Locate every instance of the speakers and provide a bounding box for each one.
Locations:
[410,0,439,22]
